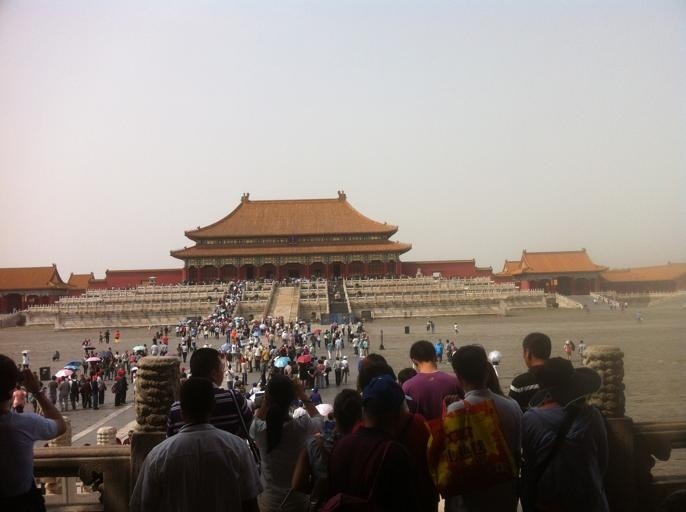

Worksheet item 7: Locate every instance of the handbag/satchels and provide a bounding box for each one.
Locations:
[34,386,46,398]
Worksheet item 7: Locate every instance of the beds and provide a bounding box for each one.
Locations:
[21,350,31,354]
[55,369,73,377]
[64,365,80,370]
[488,350,502,363]
[314,404,334,417]
[66,360,81,366]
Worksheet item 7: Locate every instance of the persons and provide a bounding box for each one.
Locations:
[220,341,245,389]
[52,350,59,361]
[198,287,245,341]
[31,392,38,413]
[47,376,58,405]
[128,375,265,512]
[22,352,29,370]
[309,388,323,405]
[570,341,576,360]
[564,340,571,360]
[245,315,369,388]
[81,329,148,377]
[402,340,465,421]
[487,360,520,408]
[522,357,610,512]
[292,389,364,512]
[443,344,525,512]
[397,367,417,385]
[0,353,67,512]
[578,340,586,365]
[248,374,324,512]
[10,385,26,414]
[166,348,253,439]
[508,332,552,413]
[447,342,457,366]
[435,339,444,363]
[446,339,450,358]
[351,363,432,448]
[327,375,440,512]
[150,319,198,363]
[36,387,47,416]
[59,378,128,411]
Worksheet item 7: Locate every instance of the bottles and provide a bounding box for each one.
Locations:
[363,374,405,400]
[529,359,601,404]
[258,374,296,422]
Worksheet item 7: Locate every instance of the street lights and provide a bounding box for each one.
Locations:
[424,396,517,498]
[321,488,366,511]
[231,388,262,475]
